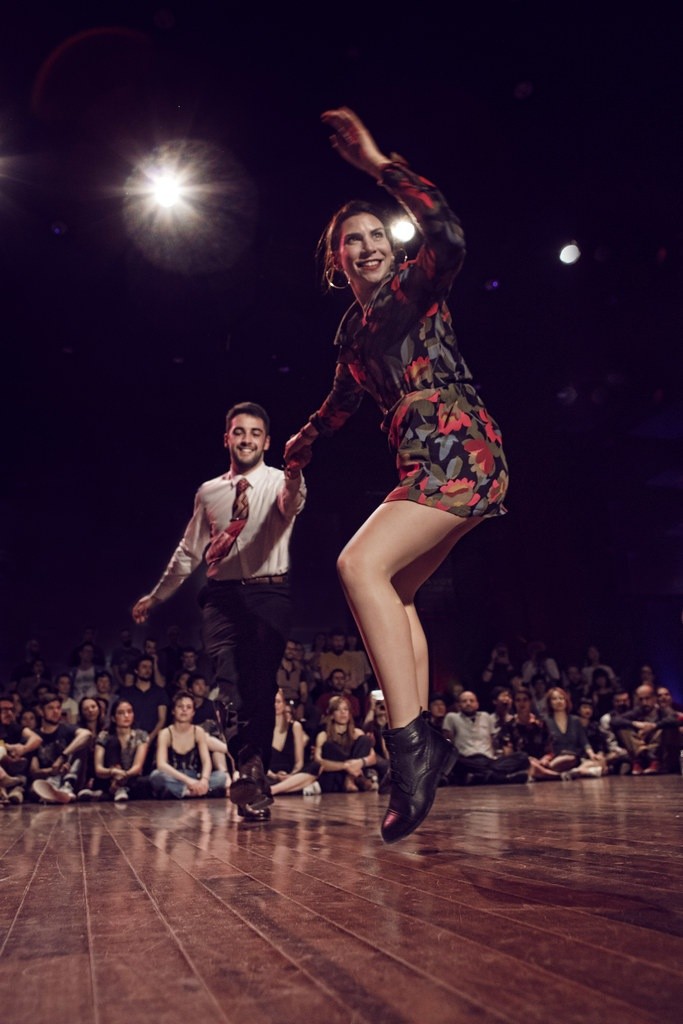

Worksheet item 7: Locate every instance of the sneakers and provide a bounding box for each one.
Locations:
[76,788,104,801]
[632,762,642,775]
[301,781,321,796]
[643,760,662,773]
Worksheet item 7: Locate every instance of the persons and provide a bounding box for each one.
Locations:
[428,646,530,785]
[147,689,227,797]
[131,402,308,820]
[119,656,169,774]
[93,697,151,802]
[124,638,170,688]
[267,633,390,798]
[0,626,120,806]
[513,639,683,782]
[164,623,241,798]
[111,630,142,685]
[285,110,509,845]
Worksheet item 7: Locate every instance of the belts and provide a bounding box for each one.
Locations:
[239,575,284,585]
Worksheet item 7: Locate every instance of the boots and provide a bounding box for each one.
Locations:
[376,710,460,844]
[229,755,275,821]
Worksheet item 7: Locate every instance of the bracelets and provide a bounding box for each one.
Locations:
[486,668,493,673]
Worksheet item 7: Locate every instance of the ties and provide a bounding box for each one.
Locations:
[205,479,250,566]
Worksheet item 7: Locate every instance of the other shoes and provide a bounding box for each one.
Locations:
[344,776,358,792]
[7,790,23,804]
[112,786,128,802]
